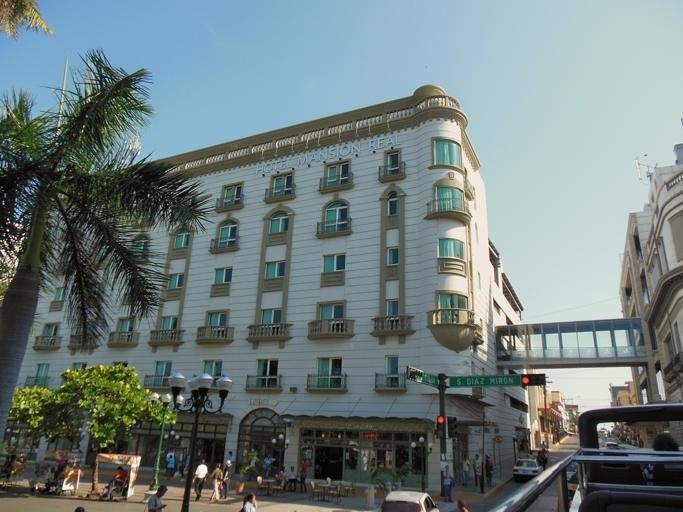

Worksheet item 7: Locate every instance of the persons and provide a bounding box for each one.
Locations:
[262,453,277,478]
[443,464,455,503]
[226,450,236,462]
[527,450,536,460]
[456,499,470,512]
[190,458,209,503]
[100,466,129,502]
[207,462,222,504]
[471,452,481,488]
[638,438,644,448]
[640,434,679,486]
[240,492,258,512]
[218,459,232,499]
[536,441,548,469]
[459,453,470,486]
[276,458,309,494]
[484,452,495,488]
[167,455,175,480]
[145,484,168,512]
[46,467,60,495]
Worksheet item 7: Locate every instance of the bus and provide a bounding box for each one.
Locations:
[491,400,683,511]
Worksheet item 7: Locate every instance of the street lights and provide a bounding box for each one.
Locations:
[163,430,181,472]
[411,436,434,492]
[169,371,234,511]
[272,433,290,479]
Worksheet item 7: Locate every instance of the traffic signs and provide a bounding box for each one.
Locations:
[445,375,522,386]
[418,370,439,389]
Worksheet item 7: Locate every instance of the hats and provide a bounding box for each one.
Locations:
[226,459,231,464]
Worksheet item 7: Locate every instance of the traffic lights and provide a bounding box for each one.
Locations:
[448,416,457,437]
[436,416,446,439]
[406,366,423,383]
[522,374,546,386]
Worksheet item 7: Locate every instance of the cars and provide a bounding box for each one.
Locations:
[513,458,544,482]
[380,491,439,512]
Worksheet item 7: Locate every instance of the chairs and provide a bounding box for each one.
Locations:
[256,476,357,504]
